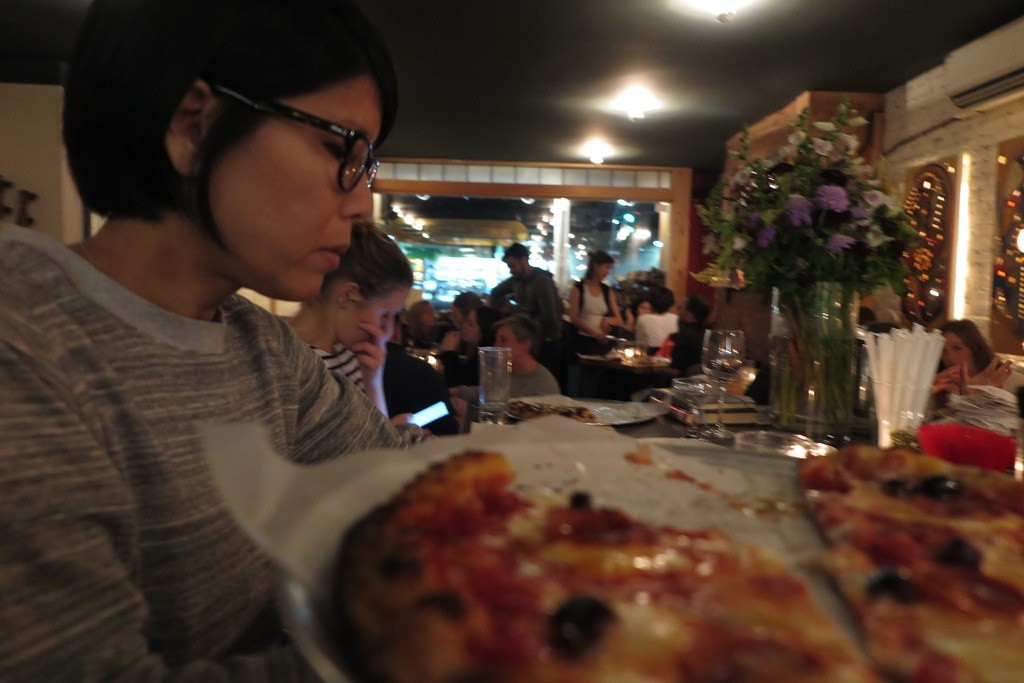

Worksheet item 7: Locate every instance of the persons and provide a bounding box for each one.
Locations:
[0,0,423,683]
[288,220,432,437]
[619,287,679,353]
[933,319,1012,396]
[569,250,622,353]
[381,291,560,438]
[491,241,562,339]
[670,295,712,378]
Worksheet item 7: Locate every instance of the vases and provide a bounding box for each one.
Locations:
[770,282,860,443]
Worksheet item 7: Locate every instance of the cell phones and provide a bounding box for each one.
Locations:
[409,402,448,428]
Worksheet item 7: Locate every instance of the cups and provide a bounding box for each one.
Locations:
[479,346,513,419]
[732,428,819,460]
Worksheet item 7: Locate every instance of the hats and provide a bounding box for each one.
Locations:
[687,296,709,318]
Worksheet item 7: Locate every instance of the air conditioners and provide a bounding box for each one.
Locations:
[945,17,1024,108]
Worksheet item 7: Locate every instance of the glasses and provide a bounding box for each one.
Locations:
[216,84,379,192]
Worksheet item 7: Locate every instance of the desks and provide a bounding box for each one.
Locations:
[580,355,670,373]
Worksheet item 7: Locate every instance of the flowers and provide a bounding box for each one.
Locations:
[694,100,920,424]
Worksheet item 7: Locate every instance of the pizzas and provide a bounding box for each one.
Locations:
[794,448,1024,683]
[508,400,597,424]
[328,445,884,683]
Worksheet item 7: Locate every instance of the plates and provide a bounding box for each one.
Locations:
[277,449,865,683]
[507,398,658,427]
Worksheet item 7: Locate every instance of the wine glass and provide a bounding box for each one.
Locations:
[673,378,712,442]
[701,328,747,440]
